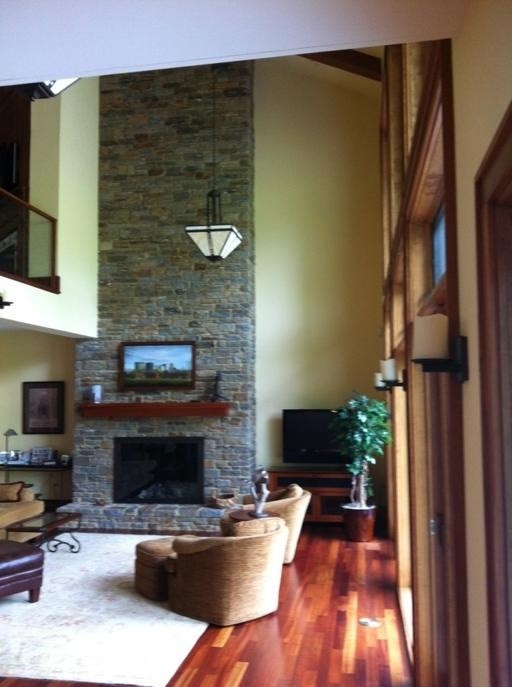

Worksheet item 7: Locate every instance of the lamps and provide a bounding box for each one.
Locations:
[3,429,18,451]
[184,65,243,263]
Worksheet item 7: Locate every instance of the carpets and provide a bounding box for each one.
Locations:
[0,533,209,687]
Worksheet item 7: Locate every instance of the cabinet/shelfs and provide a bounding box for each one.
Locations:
[79,403,233,418]
[268,464,351,523]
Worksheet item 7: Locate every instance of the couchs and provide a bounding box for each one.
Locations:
[166,517,289,627]
[0,538,44,603]
[241,484,312,563]
[0,481,45,542]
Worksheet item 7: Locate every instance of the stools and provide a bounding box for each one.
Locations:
[135,536,174,602]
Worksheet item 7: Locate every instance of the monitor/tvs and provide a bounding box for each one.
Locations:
[282,410,355,463]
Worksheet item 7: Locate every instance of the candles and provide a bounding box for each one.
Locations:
[412,314,449,359]
[379,358,397,380]
[373,372,386,387]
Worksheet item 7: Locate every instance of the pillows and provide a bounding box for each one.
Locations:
[0,481,24,502]
[278,484,304,500]
[230,518,278,535]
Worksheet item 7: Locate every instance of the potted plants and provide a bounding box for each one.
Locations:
[331,391,393,542]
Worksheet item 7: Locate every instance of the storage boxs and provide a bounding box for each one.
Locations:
[0,450,22,461]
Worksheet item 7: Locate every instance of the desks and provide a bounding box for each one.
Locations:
[0,460,72,499]
[219,509,280,536]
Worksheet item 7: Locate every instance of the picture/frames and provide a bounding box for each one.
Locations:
[22,381,65,435]
[117,340,196,391]
